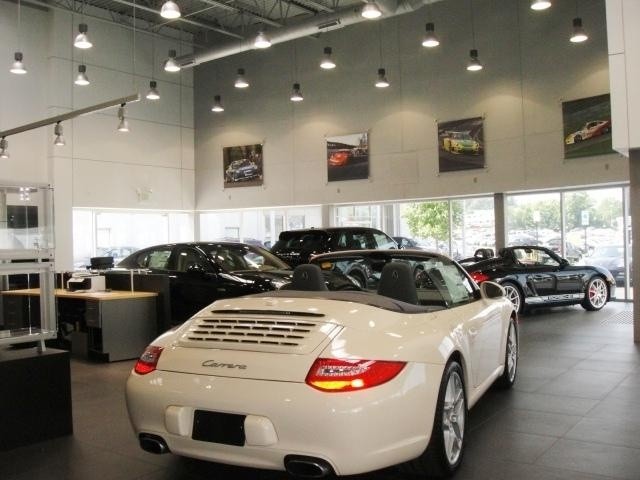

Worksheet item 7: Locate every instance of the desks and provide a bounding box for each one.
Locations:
[1,287,160,362]
[0,346,75,453]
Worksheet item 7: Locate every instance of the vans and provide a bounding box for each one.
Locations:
[268,226,408,270]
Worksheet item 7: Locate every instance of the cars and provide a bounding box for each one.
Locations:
[565,120,609,145]
[538,239,580,263]
[577,246,633,286]
[442,132,479,156]
[105,242,294,325]
[74,246,143,270]
[329,150,349,168]
[226,159,260,182]
[392,237,422,251]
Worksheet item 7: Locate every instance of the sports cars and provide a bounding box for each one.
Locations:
[125,249,518,478]
[458,245,616,314]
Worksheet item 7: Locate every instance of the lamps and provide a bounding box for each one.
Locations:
[289,41,305,103]
[362,0,382,20]
[253,27,271,50]
[420,1,441,49]
[318,45,337,71]
[0,0,225,161]
[528,0,552,14]
[464,0,484,73]
[567,1,590,45]
[372,21,391,90]
[232,68,250,90]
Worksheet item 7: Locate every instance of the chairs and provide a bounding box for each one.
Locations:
[292,263,329,292]
[376,261,420,306]
